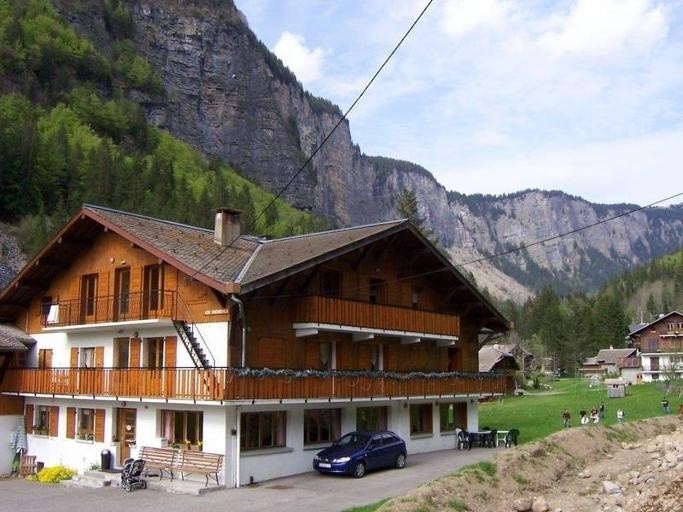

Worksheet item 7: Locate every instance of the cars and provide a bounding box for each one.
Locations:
[313,431,407,479]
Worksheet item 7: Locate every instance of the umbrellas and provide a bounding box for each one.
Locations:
[14,415,28,471]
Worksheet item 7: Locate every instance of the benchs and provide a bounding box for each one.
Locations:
[136,444,225,490]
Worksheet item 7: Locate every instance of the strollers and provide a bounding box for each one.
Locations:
[121,458,147,492]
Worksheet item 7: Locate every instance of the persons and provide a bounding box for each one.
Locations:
[591,406,600,424]
[600,401,605,418]
[616,408,624,424]
[661,397,670,413]
[580,408,589,424]
[562,409,571,427]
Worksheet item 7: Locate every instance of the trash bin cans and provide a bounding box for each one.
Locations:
[100,449,111,470]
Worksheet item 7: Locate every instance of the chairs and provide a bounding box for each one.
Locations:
[451,423,520,453]
